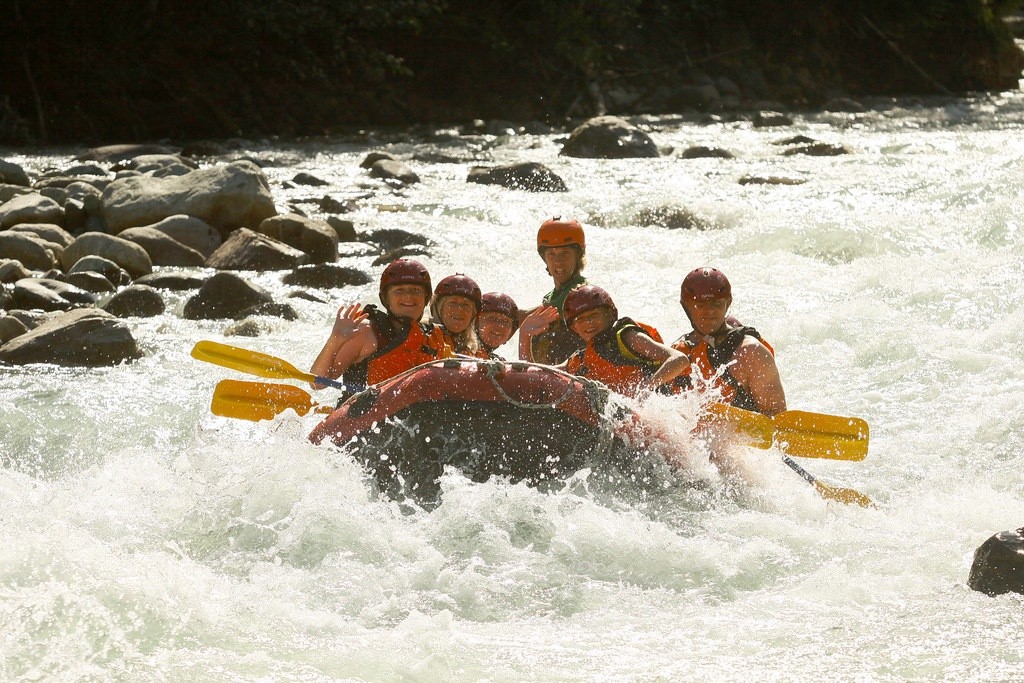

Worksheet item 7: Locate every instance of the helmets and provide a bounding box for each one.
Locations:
[562,283,618,331]
[431,273,482,318]
[380,259,432,310]
[537,216,586,263]
[475,292,520,341]
[681,267,732,312]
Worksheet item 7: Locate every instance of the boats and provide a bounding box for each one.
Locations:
[304,355,682,512]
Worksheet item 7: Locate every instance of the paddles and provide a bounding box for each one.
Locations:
[183,330,881,513]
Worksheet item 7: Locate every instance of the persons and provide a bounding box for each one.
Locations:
[309,215,691,404]
[664,267,788,421]
